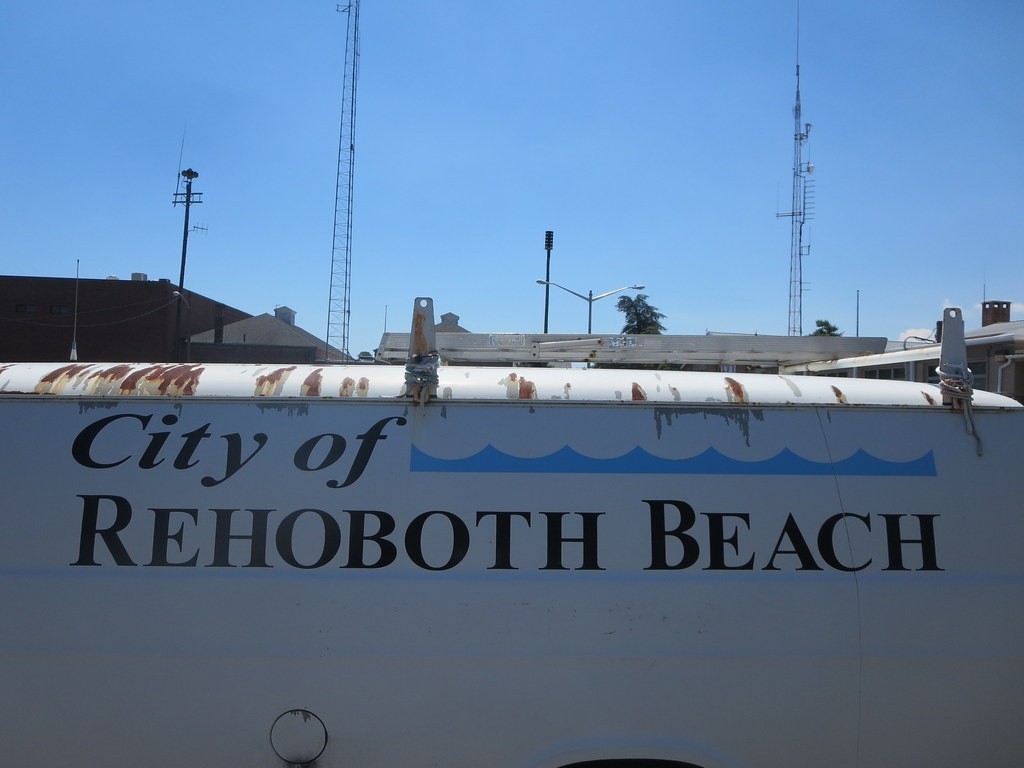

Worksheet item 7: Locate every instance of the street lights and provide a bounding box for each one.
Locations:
[534,280,646,369]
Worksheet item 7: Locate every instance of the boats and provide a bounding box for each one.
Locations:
[0,292,1023,768]
